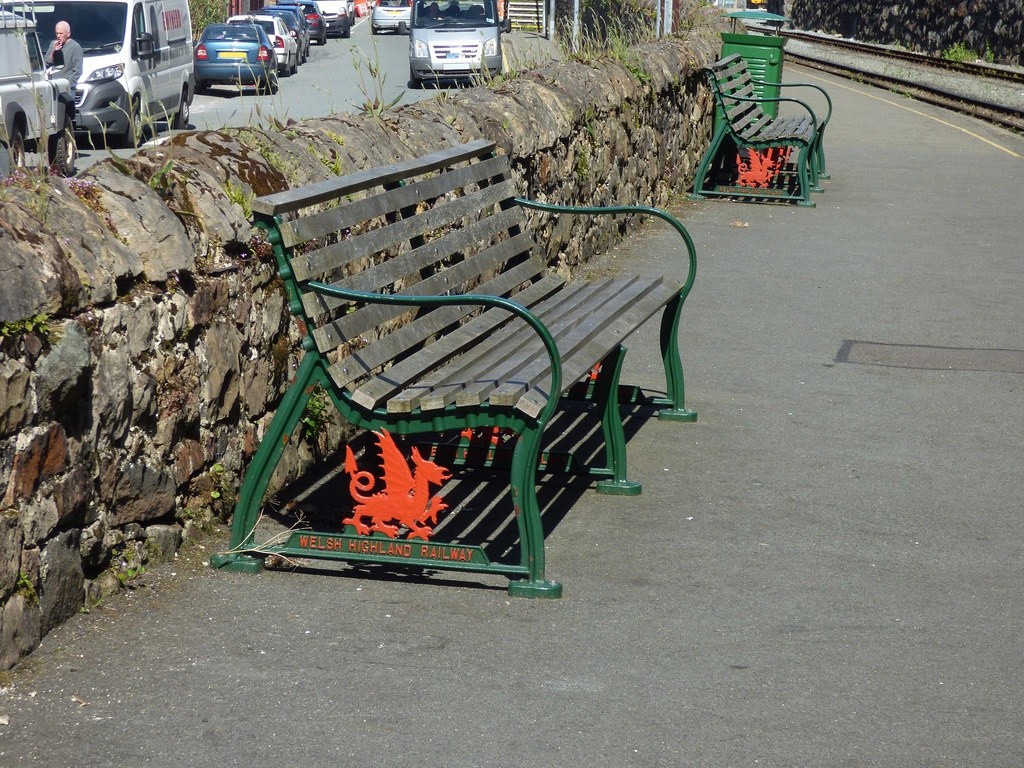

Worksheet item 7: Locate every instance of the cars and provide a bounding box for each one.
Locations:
[194,24,277,95]
[227,0,356,77]
[368,0,413,36]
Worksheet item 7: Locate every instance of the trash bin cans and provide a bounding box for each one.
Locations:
[713,9,793,160]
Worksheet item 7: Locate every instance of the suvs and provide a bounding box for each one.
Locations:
[1,1,76,177]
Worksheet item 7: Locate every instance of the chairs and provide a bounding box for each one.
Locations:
[422,6,439,17]
[467,4,485,19]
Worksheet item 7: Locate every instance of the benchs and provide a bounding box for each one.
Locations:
[209,140,697,599]
[683,52,832,209]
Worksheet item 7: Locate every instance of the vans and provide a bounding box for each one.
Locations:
[1,1,195,148]
[408,0,512,89]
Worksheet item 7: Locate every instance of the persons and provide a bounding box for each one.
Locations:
[44,20,83,103]
